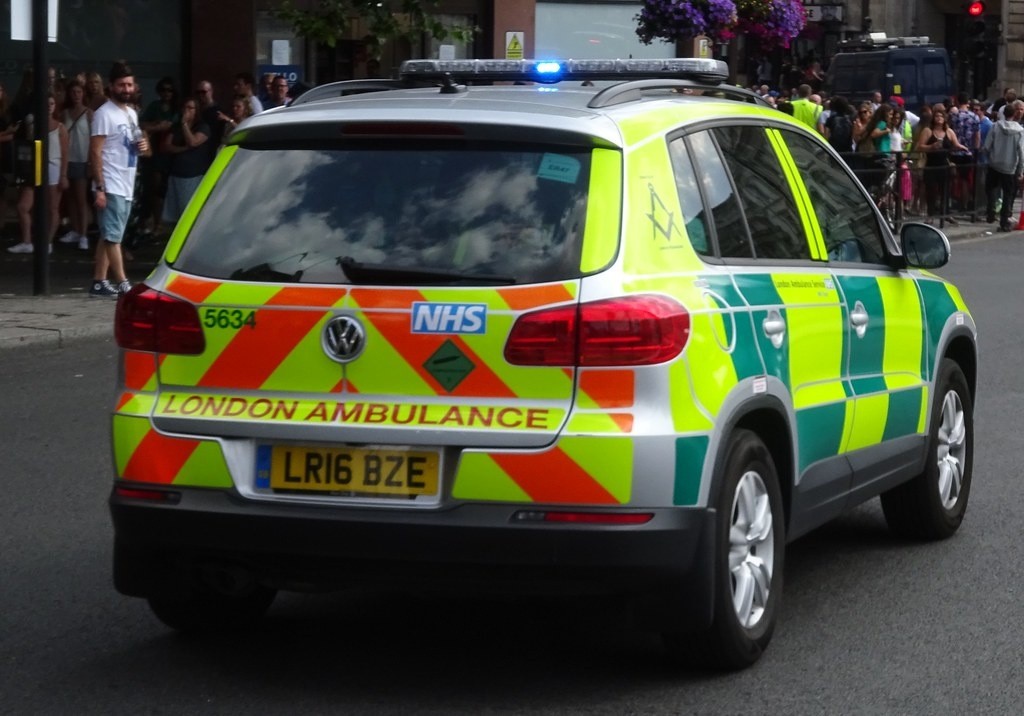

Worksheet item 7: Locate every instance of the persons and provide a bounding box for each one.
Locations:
[823,96,854,152]
[147,96,212,245]
[915,110,968,223]
[7,94,70,253]
[779,84,824,134]
[949,92,980,213]
[0,73,291,253]
[981,105,1023,232]
[852,105,873,152]
[867,106,892,149]
[87,64,151,298]
[673,53,1024,234]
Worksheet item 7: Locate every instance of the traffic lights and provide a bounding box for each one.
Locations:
[966,2,986,37]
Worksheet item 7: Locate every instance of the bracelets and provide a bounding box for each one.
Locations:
[62,175,68,177]
[96,186,105,191]
[230,119,234,122]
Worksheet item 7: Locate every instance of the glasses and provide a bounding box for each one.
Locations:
[233,92,245,98]
[194,90,208,96]
[275,83,289,88]
[185,106,196,110]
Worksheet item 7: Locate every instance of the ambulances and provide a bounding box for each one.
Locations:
[106,57,979,677]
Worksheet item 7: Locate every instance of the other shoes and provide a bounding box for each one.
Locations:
[924,219,933,225]
[1001,226,1012,231]
[987,216,997,223]
[945,216,956,224]
[883,205,1012,222]
[124,251,134,260]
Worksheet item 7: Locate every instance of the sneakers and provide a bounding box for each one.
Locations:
[60,231,80,243]
[78,237,88,249]
[8,242,34,254]
[47,244,53,253]
[89,277,134,300]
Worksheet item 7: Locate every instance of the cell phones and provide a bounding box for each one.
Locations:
[938,138,943,145]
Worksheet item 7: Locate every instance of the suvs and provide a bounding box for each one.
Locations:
[820,32,959,113]
